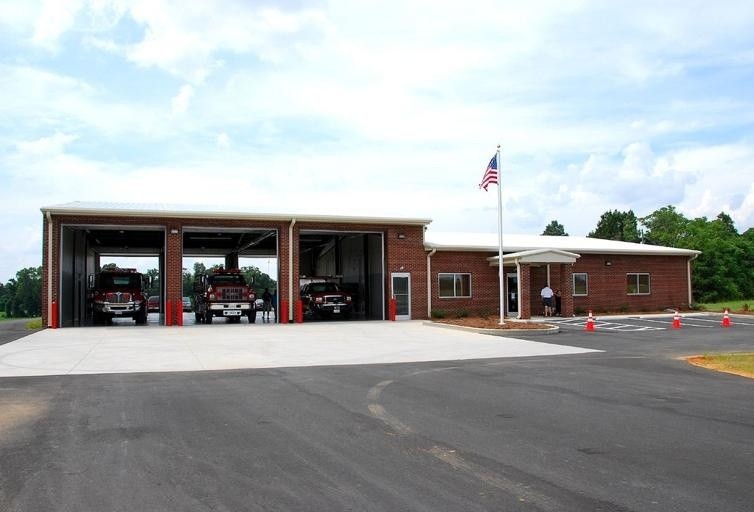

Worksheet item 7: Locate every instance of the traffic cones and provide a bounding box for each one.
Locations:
[586,309,593,331]
[722,308,729,326]
[672,310,680,329]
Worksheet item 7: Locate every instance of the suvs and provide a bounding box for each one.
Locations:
[299,282,353,321]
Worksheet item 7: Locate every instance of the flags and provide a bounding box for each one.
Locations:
[477,143,501,195]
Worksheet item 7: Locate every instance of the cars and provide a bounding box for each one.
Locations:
[147,296,160,313]
[256,299,264,311]
[182,297,192,312]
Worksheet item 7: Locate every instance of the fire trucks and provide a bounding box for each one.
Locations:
[88,268,152,325]
[194,268,256,324]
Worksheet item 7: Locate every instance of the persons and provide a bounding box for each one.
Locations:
[552,288,562,317]
[260,287,273,319]
[539,283,553,317]
[271,289,276,321]
